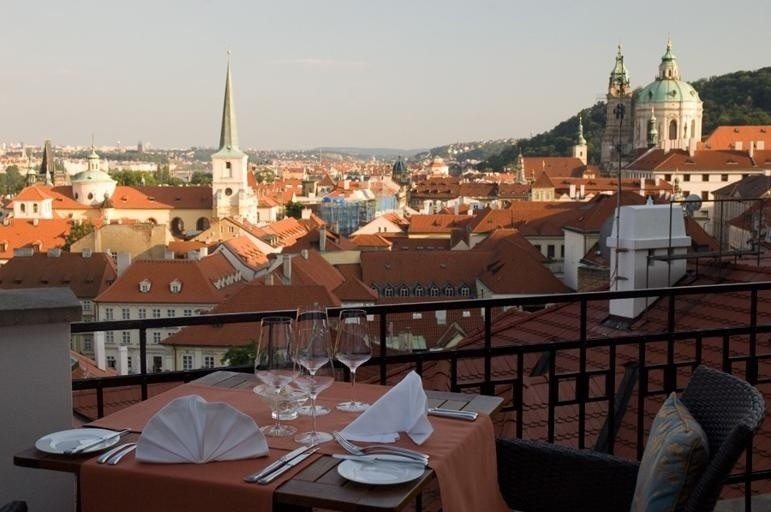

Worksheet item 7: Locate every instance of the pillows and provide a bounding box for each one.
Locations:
[630,391,710,512]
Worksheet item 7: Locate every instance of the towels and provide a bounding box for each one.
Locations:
[334,370,434,445]
[134,395,269,464]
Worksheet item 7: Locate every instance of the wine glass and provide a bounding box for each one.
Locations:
[332,309,372,412]
[255,316,297,437]
[295,302,331,417]
[290,329,334,445]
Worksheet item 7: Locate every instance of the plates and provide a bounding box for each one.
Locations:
[337,453,426,485]
[35,429,120,455]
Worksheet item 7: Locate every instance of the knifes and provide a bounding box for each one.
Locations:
[332,452,428,469]
[64,428,131,455]
[242,442,321,485]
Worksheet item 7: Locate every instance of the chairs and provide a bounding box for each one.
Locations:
[495,364,765,512]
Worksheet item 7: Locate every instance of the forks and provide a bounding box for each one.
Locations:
[331,429,430,459]
